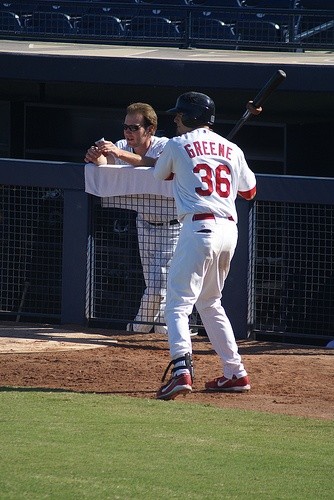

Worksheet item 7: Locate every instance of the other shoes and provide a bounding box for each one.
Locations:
[127,322,133,332]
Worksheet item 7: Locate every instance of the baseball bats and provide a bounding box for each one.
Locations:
[226,69,287,141]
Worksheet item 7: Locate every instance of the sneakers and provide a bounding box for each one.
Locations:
[155,373,192,401]
[205,375,251,391]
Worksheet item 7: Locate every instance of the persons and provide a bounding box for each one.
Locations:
[84,103,200,336]
[154,91,256,399]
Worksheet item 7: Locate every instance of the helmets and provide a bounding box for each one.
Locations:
[166,92,215,127]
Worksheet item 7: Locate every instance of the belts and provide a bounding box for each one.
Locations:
[192,213,234,221]
[147,219,179,226]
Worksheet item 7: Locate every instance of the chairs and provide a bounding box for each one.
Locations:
[0,0,334,52]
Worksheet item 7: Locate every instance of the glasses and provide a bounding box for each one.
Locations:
[122,122,145,132]
